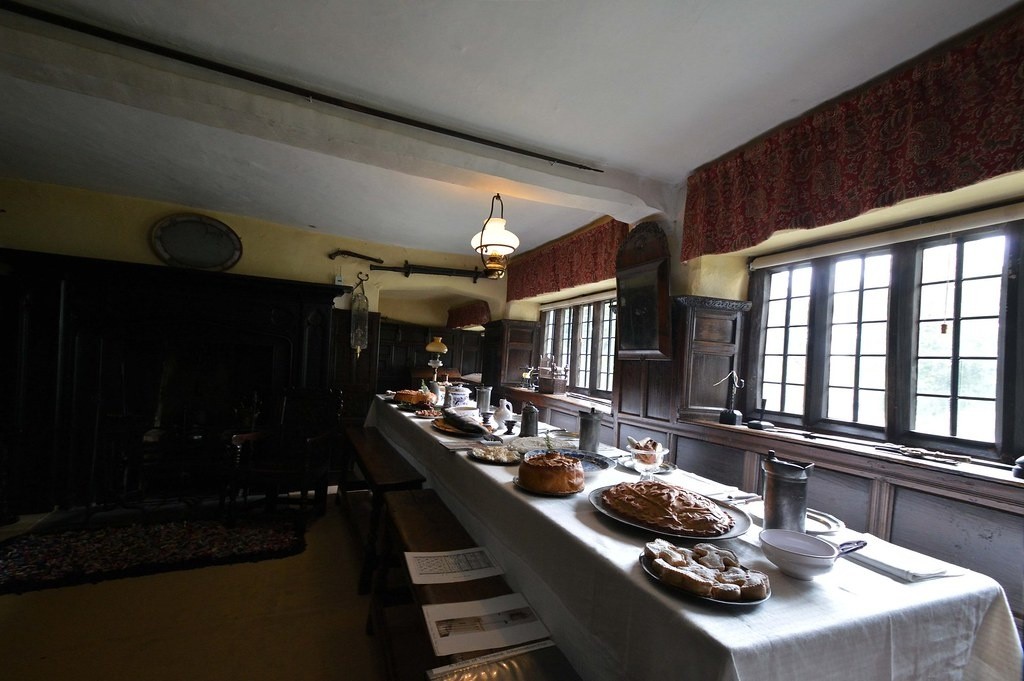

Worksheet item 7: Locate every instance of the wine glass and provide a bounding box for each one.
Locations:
[624,446,669,482]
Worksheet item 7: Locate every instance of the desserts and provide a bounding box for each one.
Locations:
[643,540,770,600]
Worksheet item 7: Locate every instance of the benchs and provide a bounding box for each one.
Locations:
[367,488,551,665]
[335,426,426,596]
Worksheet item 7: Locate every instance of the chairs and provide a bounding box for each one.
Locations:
[219,428,340,530]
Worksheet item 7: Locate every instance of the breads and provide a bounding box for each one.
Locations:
[452,407,479,419]
[519,449,584,492]
[604,481,734,534]
[394,390,418,401]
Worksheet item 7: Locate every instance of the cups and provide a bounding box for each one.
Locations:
[451,384,472,407]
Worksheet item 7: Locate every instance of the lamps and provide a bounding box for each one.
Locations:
[471,193,520,280]
[426,337,448,381]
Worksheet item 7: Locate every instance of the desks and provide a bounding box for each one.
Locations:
[364,391,1024,681]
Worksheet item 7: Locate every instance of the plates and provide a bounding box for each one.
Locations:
[588,482,753,541]
[468,450,521,464]
[513,478,582,496]
[432,418,489,437]
[617,454,679,474]
[639,547,770,606]
[752,503,846,534]
[524,448,617,478]
[414,412,444,419]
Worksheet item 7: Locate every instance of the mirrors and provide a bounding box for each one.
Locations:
[615,221,674,362]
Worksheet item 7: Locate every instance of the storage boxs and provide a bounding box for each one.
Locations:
[540,377,567,395]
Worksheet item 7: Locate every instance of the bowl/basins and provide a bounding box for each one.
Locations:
[759,528,837,582]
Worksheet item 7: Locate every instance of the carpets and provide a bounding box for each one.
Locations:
[0,506,311,596]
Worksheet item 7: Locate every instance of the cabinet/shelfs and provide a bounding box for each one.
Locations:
[460,378,1024,650]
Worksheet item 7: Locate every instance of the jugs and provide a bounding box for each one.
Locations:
[578,407,603,453]
[493,399,513,428]
[475,383,493,413]
[757,449,814,535]
[519,401,539,437]
